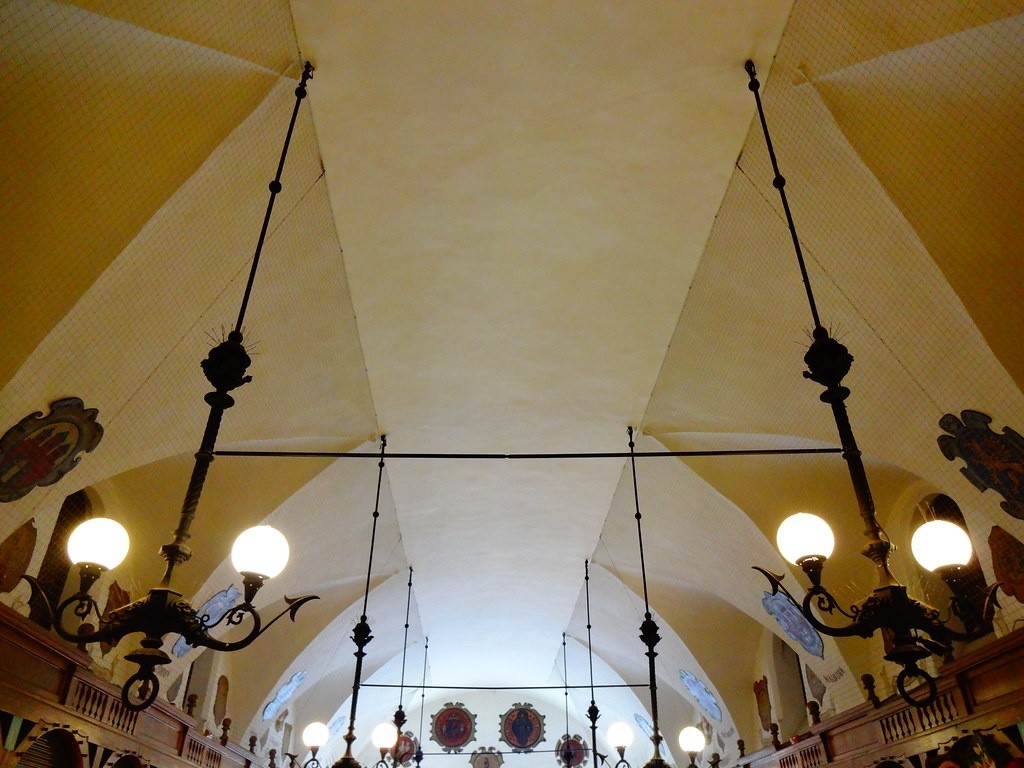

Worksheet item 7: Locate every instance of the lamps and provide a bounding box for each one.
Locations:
[22,63,316,709]
[742,58,1006,708]
[604,425,707,768]
[285,432,399,768]
